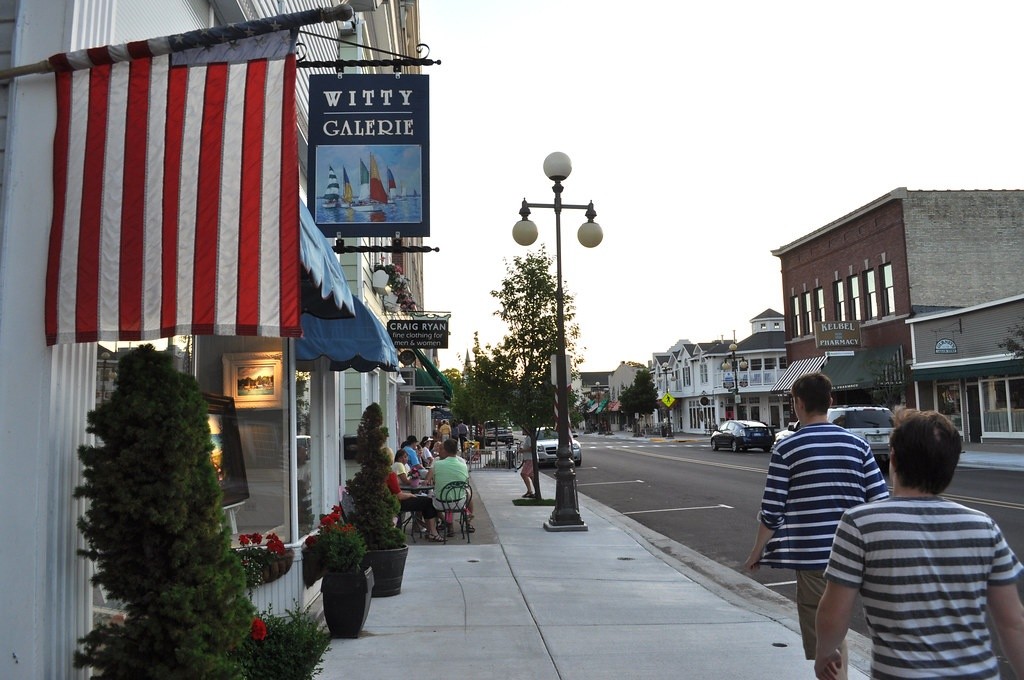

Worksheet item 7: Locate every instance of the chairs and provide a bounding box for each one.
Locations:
[395,482,475,544]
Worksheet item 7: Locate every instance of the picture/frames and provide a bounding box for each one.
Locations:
[202,392,249,508]
[223,350,283,411]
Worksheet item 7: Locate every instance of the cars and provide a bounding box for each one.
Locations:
[710,419,776,453]
[467,426,480,435]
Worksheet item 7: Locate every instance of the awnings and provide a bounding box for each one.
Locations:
[771,345,906,390]
[299,196,399,374]
[430,408,453,420]
[409,349,453,407]
[586,399,622,414]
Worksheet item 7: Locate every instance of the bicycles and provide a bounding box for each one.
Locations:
[465,435,475,465]
[513,442,525,469]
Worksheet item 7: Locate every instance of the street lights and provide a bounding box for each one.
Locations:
[657,363,678,438]
[722,344,748,419]
[591,381,605,434]
[512,151,603,528]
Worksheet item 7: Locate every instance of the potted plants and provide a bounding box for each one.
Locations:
[321,403,410,639]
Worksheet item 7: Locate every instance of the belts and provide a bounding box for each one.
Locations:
[436,498,462,503]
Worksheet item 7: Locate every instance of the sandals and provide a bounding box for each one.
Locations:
[428,534,448,542]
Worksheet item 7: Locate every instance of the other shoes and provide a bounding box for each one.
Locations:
[522,491,536,498]
[466,525,475,532]
[448,523,454,537]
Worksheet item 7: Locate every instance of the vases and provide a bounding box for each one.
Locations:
[302,545,329,589]
[383,291,401,313]
[244,550,295,589]
[410,478,420,487]
[373,269,392,296]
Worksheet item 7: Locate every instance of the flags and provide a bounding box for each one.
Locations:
[43,6,329,345]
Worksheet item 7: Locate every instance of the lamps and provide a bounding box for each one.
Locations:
[395,370,406,384]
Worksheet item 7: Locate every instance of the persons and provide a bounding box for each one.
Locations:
[519,424,536,497]
[814,406,1024,680]
[746,372,889,680]
[381,419,475,542]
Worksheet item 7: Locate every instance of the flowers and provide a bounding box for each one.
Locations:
[234,532,287,595]
[375,262,418,315]
[305,507,358,546]
[408,467,421,480]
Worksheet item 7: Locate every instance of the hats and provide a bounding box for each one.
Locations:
[408,436,419,442]
[432,442,441,450]
[424,437,434,443]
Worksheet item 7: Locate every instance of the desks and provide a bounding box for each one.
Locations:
[399,484,435,539]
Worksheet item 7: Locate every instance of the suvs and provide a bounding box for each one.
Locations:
[775,406,898,473]
[534,426,582,465]
[482,419,514,444]
